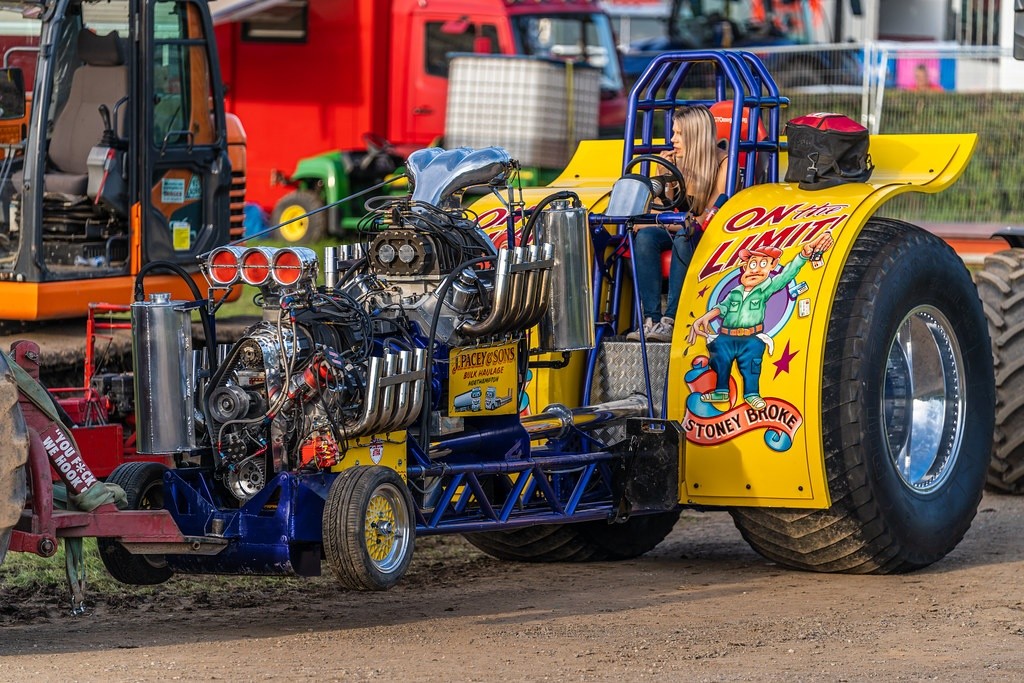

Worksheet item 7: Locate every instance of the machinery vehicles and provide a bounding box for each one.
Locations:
[0,50,996,577]
[617,0,861,86]
[0,0,248,403]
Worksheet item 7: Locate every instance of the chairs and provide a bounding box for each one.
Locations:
[621,98,762,280]
[12,65,133,197]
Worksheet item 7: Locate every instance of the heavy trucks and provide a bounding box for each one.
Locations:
[209,0,629,220]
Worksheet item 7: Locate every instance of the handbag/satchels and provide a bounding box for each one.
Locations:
[781,112,876,191]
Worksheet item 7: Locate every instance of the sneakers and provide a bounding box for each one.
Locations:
[647,317,674,342]
[625,317,657,342]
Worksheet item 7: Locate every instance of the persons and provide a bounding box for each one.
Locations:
[626,104,740,343]
[908,64,944,92]
